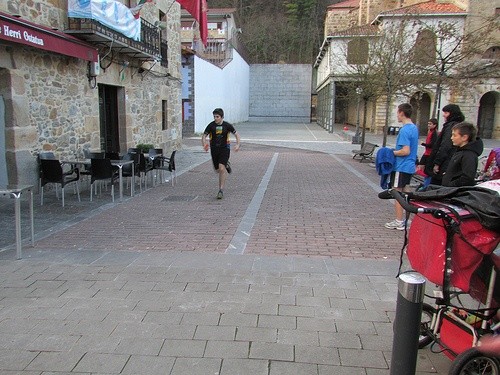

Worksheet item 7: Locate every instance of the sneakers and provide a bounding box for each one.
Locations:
[384,218,411,231]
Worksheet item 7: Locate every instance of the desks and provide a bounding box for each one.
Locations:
[0,184,35,260]
[61,158,135,201]
[143,153,164,187]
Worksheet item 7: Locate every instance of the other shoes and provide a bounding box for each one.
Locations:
[226,161,232,174]
[216,191,223,199]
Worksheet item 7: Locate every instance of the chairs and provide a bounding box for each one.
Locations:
[38,151,81,208]
[80,148,177,202]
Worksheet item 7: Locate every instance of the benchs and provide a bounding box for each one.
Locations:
[352,142,379,163]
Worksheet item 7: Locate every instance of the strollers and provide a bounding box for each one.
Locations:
[377,179,500,375]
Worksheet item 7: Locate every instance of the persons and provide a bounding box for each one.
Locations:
[202,108,240,199]
[416,103,483,192]
[376,103,418,230]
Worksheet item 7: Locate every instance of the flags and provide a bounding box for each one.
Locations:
[130,0,152,19]
[176,0,208,46]
